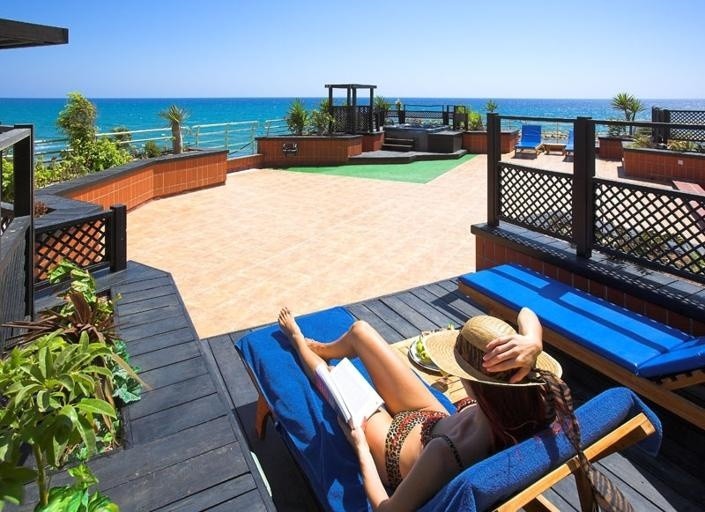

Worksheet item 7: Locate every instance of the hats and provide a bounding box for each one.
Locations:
[423,315,563,387]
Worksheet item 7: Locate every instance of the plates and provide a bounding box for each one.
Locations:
[408,334,443,373]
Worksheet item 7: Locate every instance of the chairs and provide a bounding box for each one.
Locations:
[459,261,704,431]
[563,128,575,162]
[233,305,663,512]
[514,124,544,158]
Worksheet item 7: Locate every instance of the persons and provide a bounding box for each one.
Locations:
[277,305,563,511]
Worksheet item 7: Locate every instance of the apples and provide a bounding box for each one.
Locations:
[417,340,424,353]
[420,351,432,364]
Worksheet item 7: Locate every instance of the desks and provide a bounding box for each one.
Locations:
[543,142,566,157]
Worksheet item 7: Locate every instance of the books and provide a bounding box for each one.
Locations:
[314,357,385,431]
